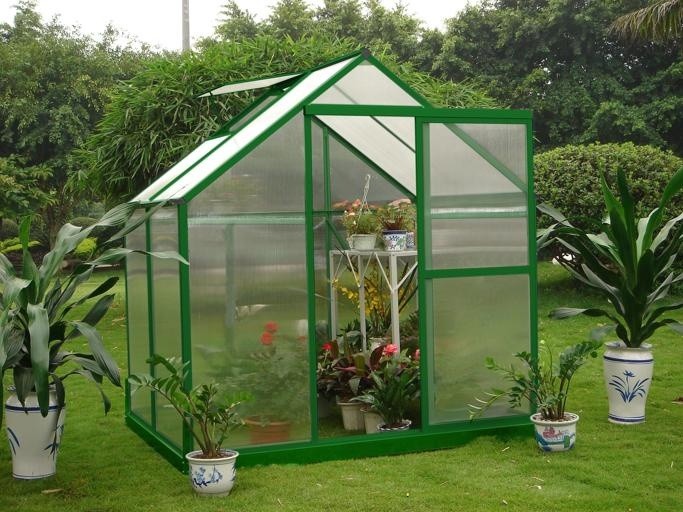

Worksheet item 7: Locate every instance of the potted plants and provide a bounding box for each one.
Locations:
[375,200,416,252]
[536,155,683,426]
[196,342,309,444]
[466,318,623,452]
[0,182,191,481]
[128,354,256,497]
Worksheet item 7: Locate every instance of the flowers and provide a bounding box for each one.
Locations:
[321,243,418,337]
[261,323,420,426]
[338,199,384,235]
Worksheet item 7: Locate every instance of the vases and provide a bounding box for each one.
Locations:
[338,401,411,435]
[348,232,378,250]
